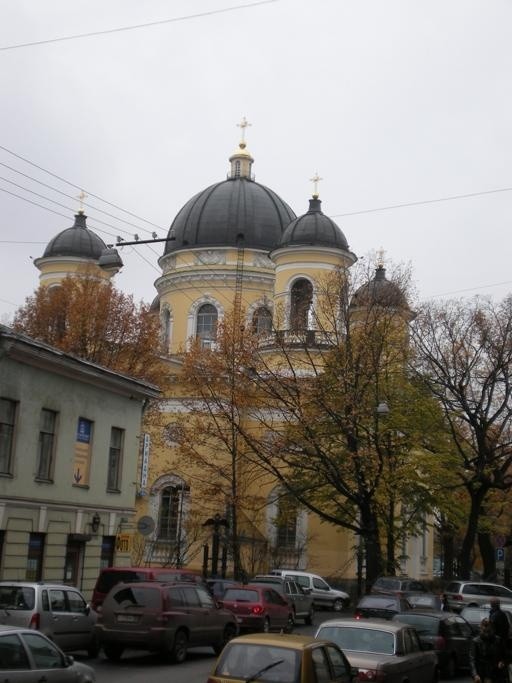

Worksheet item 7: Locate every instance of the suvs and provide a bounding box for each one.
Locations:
[272,569,349,611]
[354,575,512,675]
[0,582,102,658]
[95,580,239,662]
[247,575,315,624]
[207,632,352,682]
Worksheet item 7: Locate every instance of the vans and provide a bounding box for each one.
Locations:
[92,567,207,612]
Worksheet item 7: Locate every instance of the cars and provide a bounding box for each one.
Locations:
[204,578,238,604]
[0,624,96,682]
[315,617,439,682]
[218,585,294,632]
